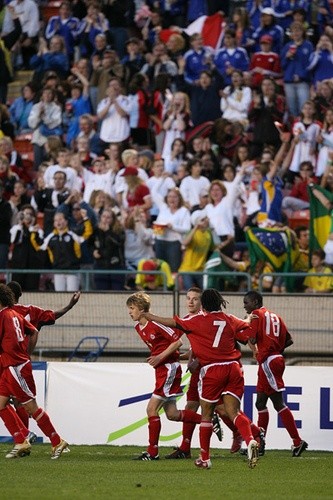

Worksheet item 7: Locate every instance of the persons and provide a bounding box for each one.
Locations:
[127,287,309,470]
[1,0,333,293]
[0,278,81,460]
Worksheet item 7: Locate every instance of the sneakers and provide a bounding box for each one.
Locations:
[231,430,244,452]
[133,452,160,461]
[4,440,32,459]
[254,427,265,456]
[50,439,68,460]
[194,455,212,469]
[211,412,223,442]
[165,447,191,459]
[20,431,38,457]
[247,440,258,468]
[292,441,308,457]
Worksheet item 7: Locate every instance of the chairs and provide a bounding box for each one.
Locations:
[68,336,109,362]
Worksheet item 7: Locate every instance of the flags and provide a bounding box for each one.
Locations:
[306,180,333,262]
[160,14,228,49]
[243,225,297,270]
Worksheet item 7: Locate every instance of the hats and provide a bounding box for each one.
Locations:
[120,168,137,178]
[259,36,272,43]
[103,44,115,54]
[125,38,139,45]
[261,7,276,16]
